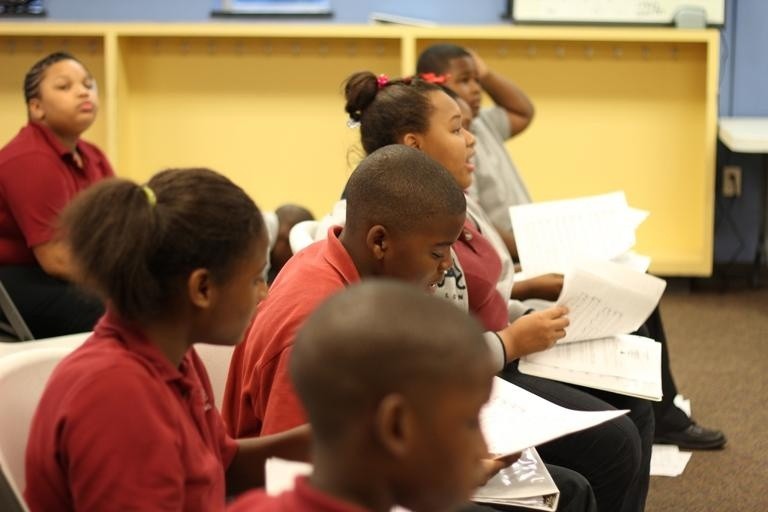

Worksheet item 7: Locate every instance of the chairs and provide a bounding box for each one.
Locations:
[0,335,108,508]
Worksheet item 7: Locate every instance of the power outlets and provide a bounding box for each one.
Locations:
[724,166,741,198]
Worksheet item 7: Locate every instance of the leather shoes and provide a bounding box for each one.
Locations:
[654,420,726,449]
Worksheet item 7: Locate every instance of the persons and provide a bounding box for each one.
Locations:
[440,83,650,340]
[270,204,314,281]
[0,51,116,341]
[220,145,596,511]
[226,277,486,511]
[316,72,657,512]
[419,45,727,450]
[25,167,311,512]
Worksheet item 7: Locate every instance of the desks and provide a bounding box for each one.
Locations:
[719,116,766,287]
[1,0,721,289]
